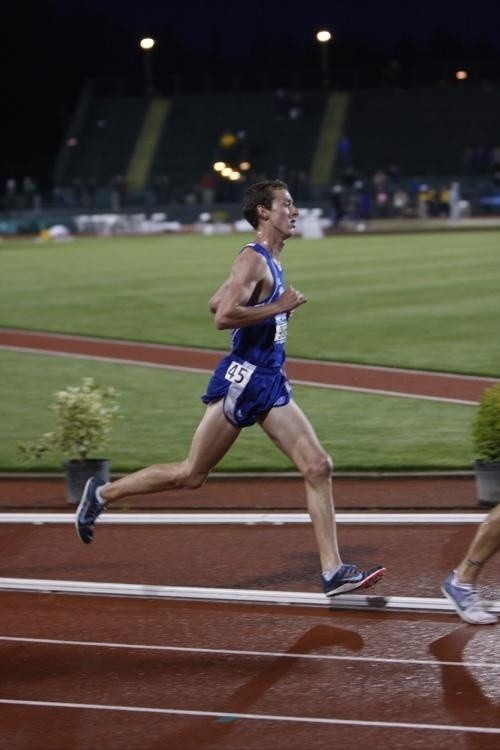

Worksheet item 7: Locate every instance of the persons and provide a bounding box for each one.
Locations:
[441,504,499,625]
[75,179,386,598]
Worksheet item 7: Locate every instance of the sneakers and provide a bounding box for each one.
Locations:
[320,564,386,597]
[440,571,498,624]
[75,476,106,544]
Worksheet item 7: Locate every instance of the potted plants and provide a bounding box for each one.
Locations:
[18,376,124,505]
[471,382,500,505]
